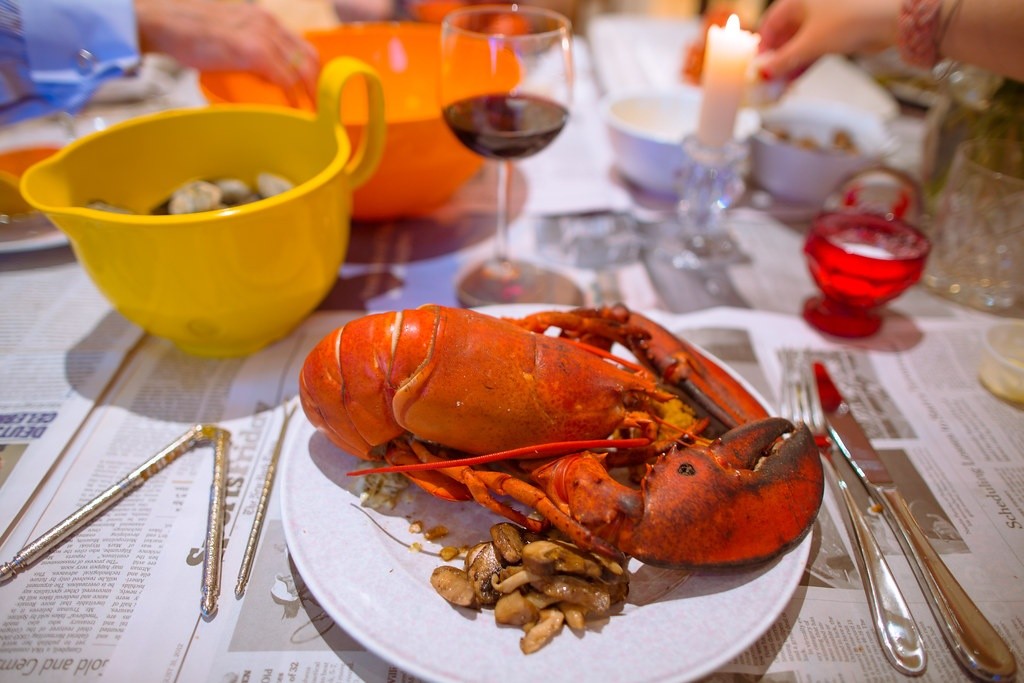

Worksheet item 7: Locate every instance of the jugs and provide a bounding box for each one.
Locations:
[18,55,385,357]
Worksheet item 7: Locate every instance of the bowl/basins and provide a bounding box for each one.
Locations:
[805,210,932,339]
[600,86,766,205]
[751,137,856,207]
[194,15,520,220]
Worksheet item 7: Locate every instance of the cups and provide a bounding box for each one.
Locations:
[923,140,1024,317]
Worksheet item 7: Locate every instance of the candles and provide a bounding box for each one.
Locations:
[697,13,762,149]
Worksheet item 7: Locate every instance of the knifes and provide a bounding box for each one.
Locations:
[811,358,1019,683]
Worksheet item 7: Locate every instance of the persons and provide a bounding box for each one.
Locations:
[0,0,325,126]
[757,0,1024,83]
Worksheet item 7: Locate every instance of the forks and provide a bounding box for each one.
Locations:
[780,381,928,676]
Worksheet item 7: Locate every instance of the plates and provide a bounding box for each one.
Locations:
[1,112,144,249]
[279,303,816,683]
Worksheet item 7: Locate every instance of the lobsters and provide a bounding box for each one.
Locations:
[299,302,826,572]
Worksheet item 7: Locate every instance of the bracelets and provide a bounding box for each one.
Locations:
[893,0,941,69]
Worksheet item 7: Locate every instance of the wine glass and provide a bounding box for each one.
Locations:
[441,8,575,309]
